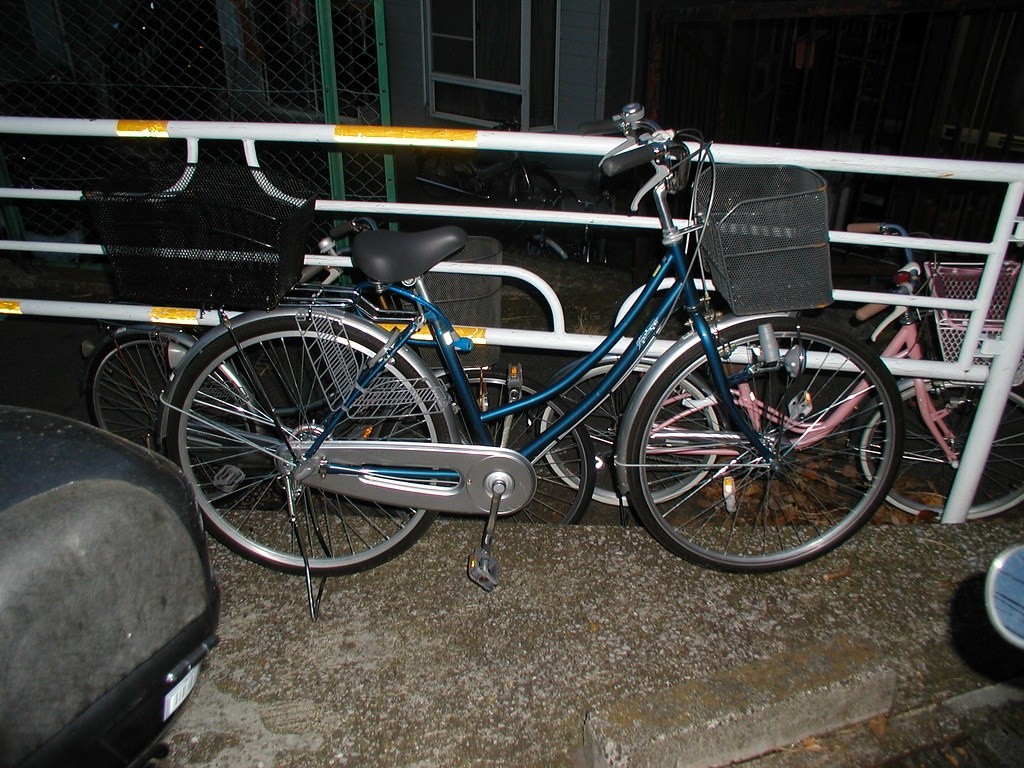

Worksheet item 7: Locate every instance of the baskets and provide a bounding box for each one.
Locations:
[400,235,500,368]
[82,160,319,314]
[924,261,1024,387]
[686,163,831,314]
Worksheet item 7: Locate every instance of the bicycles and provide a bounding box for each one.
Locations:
[91,101,1024,620]
